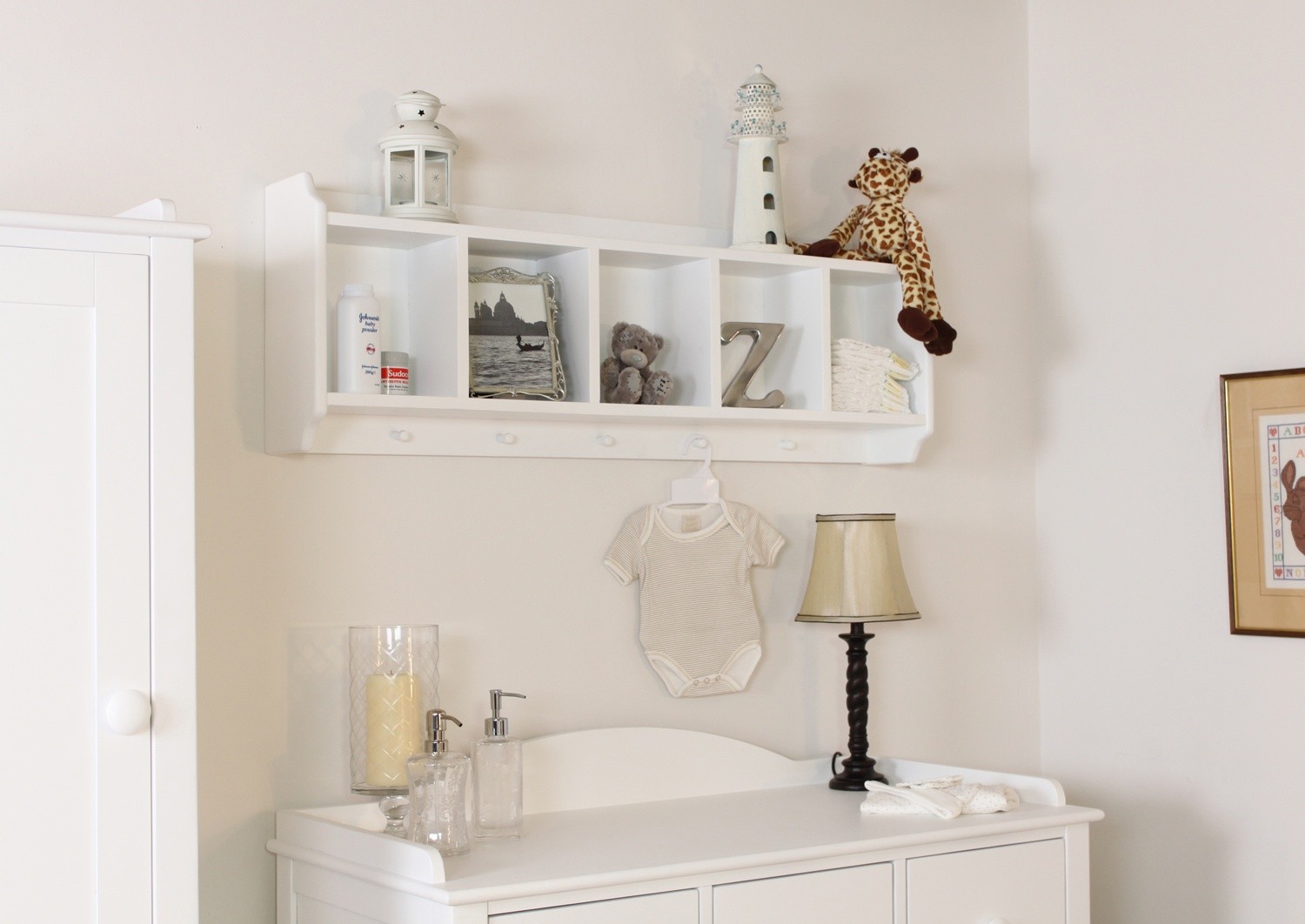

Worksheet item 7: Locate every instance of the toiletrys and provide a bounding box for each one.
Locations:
[337,283,409,396]
[474,689,527,840]
[406,708,472,859]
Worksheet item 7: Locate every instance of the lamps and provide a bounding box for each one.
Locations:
[794,512,922,792]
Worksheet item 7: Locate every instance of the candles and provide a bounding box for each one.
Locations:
[368,672,424,784]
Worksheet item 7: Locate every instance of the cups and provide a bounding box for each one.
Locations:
[349,624,440,795]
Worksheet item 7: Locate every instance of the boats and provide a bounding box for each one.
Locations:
[517,339,545,351]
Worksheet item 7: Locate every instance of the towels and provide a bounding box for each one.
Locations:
[830,337,920,415]
[858,773,1021,820]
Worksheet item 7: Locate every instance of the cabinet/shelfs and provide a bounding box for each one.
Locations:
[266,771,1106,924]
[0,200,212,924]
[265,173,937,464]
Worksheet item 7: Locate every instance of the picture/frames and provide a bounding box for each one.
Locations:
[467,268,567,402]
[1217,368,1305,638]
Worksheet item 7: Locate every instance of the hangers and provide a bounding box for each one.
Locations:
[654,435,724,517]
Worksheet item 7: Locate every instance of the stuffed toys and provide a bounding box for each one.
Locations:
[600,322,674,404]
[787,146,957,356]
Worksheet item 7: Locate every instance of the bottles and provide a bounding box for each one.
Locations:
[336,283,382,397]
[381,351,412,395]
